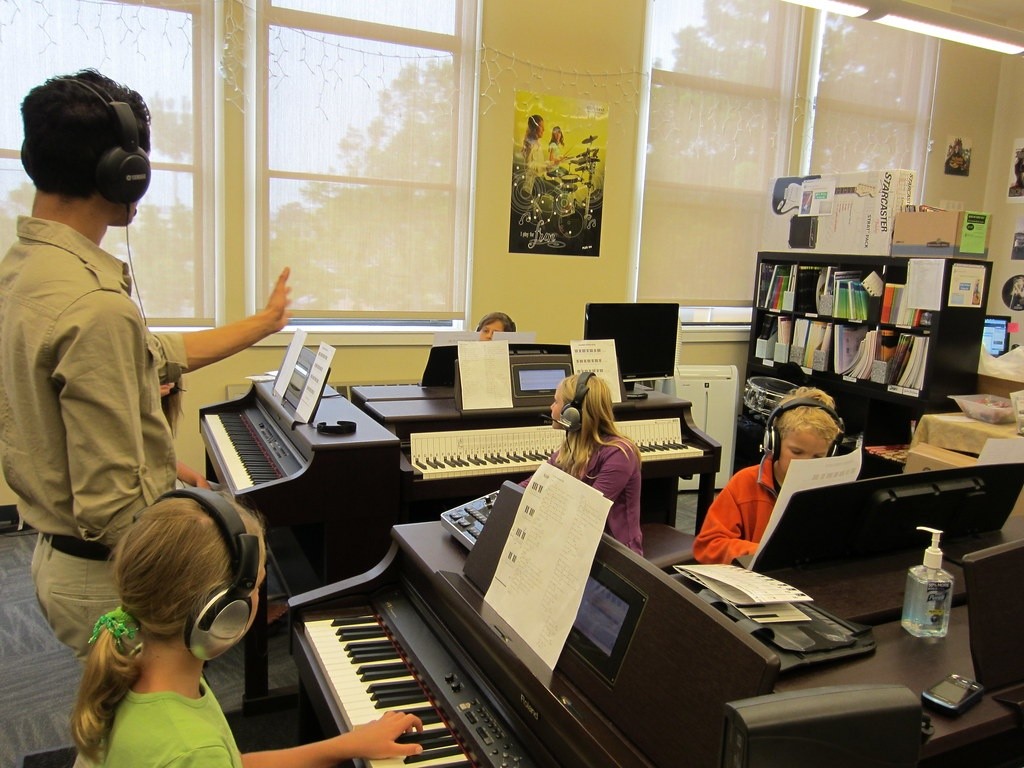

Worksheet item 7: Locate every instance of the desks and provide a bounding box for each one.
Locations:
[922,412,1024,453]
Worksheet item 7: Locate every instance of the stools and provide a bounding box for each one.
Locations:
[638,522,697,570]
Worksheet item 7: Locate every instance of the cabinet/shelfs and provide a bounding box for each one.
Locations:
[740,250,994,457]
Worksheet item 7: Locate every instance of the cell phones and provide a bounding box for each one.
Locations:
[922,672,985,718]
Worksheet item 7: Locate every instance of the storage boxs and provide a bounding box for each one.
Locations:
[891,211,993,258]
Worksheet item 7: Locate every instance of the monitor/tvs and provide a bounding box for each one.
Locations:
[981,315,1011,358]
[584,303,679,398]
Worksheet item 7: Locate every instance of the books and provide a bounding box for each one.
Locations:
[901,204,948,212]
[759,258,946,391]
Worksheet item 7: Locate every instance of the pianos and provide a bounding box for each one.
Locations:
[668,460,1024,623]
[338,343,722,539]
[199,341,400,694]
[287,481,781,768]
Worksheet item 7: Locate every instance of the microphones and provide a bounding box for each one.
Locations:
[541,415,571,429]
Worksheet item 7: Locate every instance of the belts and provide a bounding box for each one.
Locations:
[45,533,111,561]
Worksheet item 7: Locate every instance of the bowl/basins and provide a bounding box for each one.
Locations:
[947,393,1016,425]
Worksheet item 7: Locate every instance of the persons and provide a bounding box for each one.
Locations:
[514,372,643,556]
[0,73,292,668]
[471,311,515,341]
[690,387,842,564]
[522,114,565,179]
[158,374,211,491]
[70,488,423,768]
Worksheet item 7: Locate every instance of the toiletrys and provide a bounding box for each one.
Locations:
[901,526,955,639]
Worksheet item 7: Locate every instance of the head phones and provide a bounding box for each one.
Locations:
[763,397,846,460]
[559,372,597,431]
[56,77,151,205]
[152,487,260,660]
